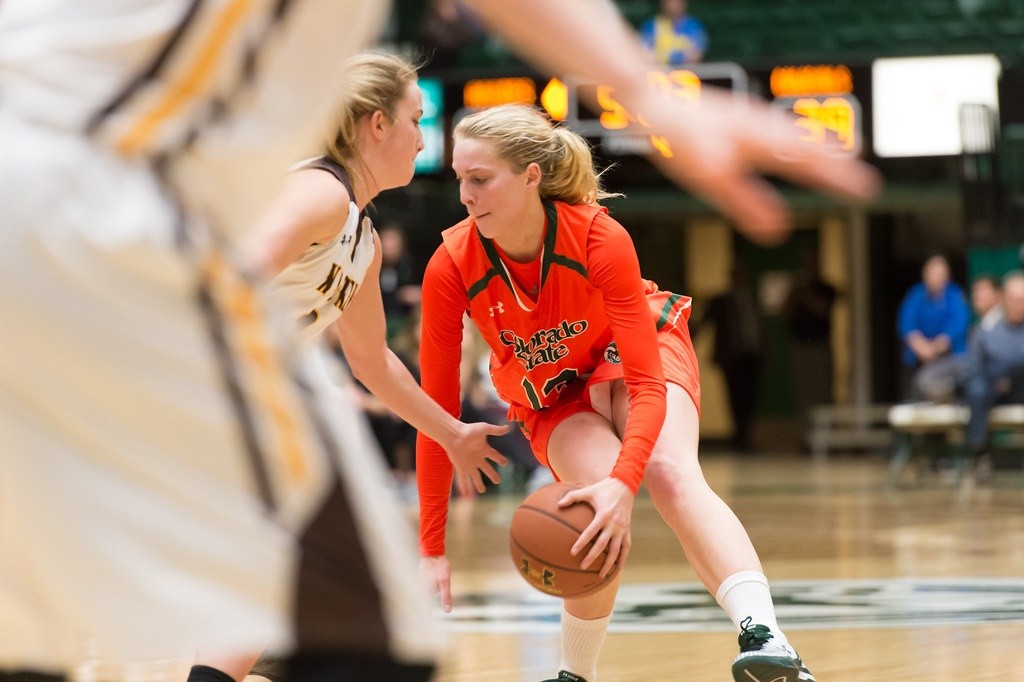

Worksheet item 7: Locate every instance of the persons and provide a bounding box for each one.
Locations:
[888,253,1024,474]
[1,0,878,682]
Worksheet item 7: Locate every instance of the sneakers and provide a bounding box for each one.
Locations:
[731,617,816,682]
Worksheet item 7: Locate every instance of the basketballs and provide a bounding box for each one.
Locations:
[514,479,626,601]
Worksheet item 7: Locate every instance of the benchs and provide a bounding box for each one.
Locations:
[889,401,1024,473]
